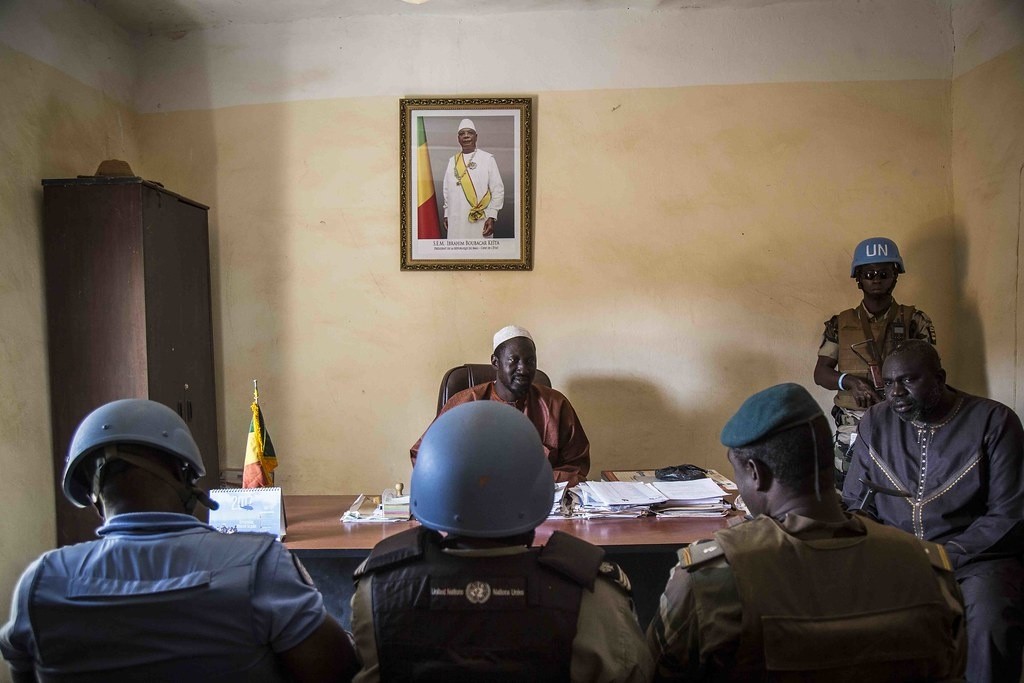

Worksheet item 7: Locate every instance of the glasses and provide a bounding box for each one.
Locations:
[859,269,896,281]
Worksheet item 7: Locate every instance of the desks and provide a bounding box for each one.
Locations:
[191,491,746,558]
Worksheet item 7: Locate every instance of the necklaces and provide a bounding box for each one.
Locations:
[454,150,477,185]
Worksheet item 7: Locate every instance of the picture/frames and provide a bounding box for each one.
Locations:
[400,98,532,272]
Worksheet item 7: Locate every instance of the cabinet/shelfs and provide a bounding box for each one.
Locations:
[42,177,220,549]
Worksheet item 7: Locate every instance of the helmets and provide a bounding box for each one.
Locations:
[61,399,206,508]
[850,238,905,278]
[409,400,555,538]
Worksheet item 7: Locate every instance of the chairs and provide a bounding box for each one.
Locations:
[437,364,552,415]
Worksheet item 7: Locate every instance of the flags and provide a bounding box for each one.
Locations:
[417,117,441,239]
[242,404,277,488]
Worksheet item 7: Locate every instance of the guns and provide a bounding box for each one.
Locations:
[850,338,888,402]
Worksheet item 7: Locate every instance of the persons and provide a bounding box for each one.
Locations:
[841,339,1024,683]
[648,383,969,683]
[443,119,504,238]
[410,326,591,486]
[354,401,657,683]
[0,399,355,683]
[813,237,935,496]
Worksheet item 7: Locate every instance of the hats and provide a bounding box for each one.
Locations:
[492,325,536,355]
[458,119,476,133]
[721,383,824,447]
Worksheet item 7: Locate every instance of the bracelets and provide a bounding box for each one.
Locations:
[838,374,849,390]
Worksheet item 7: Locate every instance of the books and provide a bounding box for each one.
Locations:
[548,477,732,519]
